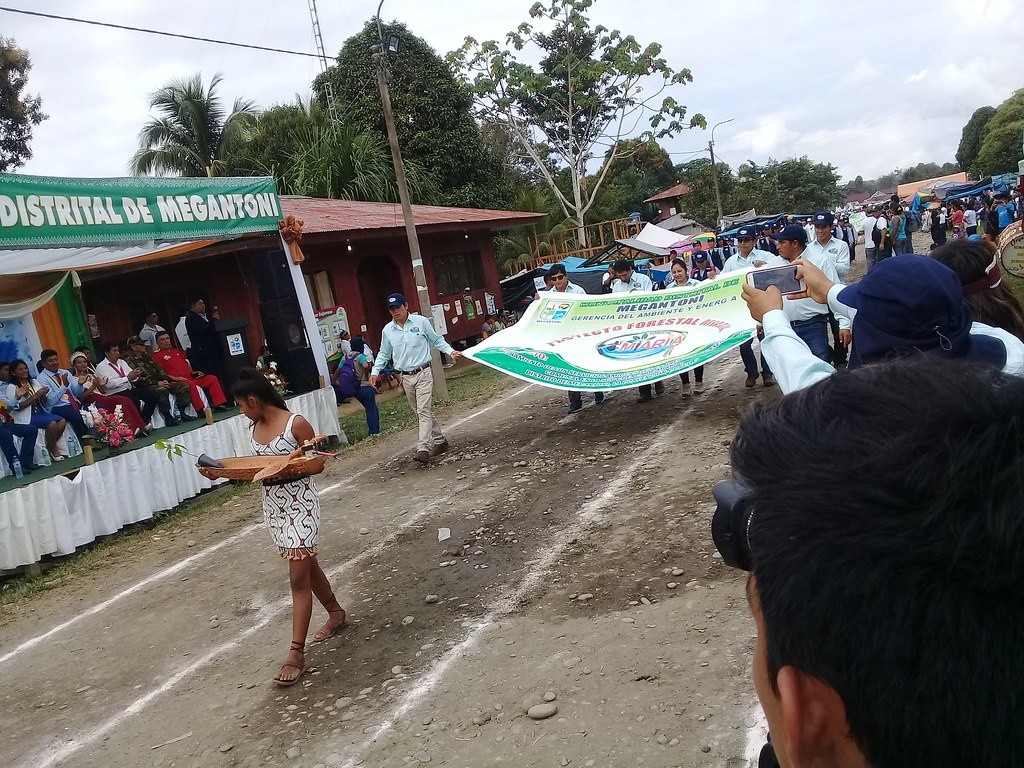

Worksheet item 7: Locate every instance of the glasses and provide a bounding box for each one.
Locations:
[551,273,566,281]
[738,238,755,243]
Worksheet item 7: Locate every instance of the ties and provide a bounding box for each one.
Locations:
[54,373,78,410]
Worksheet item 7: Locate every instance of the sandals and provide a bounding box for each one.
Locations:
[274,641,306,686]
[313,592,347,642]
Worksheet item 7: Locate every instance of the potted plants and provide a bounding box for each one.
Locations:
[154,438,224,468]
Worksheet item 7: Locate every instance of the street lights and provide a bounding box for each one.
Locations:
[708,117,736,226]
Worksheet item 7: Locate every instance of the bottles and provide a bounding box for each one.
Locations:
[42,445,51,465]
[13,455,23,478]
[68,435,80,457]
[174,405,181,421]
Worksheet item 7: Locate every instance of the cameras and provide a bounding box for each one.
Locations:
[712,480,759,572]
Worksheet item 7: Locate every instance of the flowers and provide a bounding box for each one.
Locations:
[258,362,294,395]
[79,401,134,449]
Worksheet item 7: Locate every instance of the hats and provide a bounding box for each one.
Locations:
[736,226,756,238]
[772,223,779,229]
[695,253,706,262]
[836,252,1007,374]
[692,240,701,247]
[762,222,770,230]
[386,293,405,309]
[716,236,723,241]
[126,335,148,348]
[707,237,714,243]
[769,224,807,245]
[813,211,835,225]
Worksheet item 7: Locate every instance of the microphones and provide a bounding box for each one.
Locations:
[214,306,218,312]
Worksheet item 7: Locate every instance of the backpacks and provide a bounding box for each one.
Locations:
[339,353,362,397]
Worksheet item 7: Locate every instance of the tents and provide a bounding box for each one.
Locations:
[854,171,1019,209]
[498,208,825,302]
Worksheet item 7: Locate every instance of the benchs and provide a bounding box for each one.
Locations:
[0,385,211,479]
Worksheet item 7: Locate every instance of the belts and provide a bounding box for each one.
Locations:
[790,314,827,327]
[402,362,432,374]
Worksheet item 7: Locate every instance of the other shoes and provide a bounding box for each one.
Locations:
[428,439,449,457]
[12,401,242,476]
[412,451,430,464]
[566,371,775,414]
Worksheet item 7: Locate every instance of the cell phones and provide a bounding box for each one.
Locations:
[746,264,807,295]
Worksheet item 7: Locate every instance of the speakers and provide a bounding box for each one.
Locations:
[255,250,320,396]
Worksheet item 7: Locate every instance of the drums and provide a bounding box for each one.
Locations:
[997,219,1024,277]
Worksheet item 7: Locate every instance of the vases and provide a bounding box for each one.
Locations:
[105,442,119,456]
[277,391,283,399]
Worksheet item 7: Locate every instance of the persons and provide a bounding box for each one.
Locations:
[0,311,232,478]
[195,368,351,686]
[765,210,853,372]
[531,264,605,413]
[612,261,665,404]
[481,308,507,341]
[186,296,228,408]
[368,293,461,464]
[742,258,1024,394]
[669,192,1024,281]
[667,260,706,397]
[927,240,1024,338]
[335,330,381,437]
[733,358,1024,768]
[707,229,792,389]
